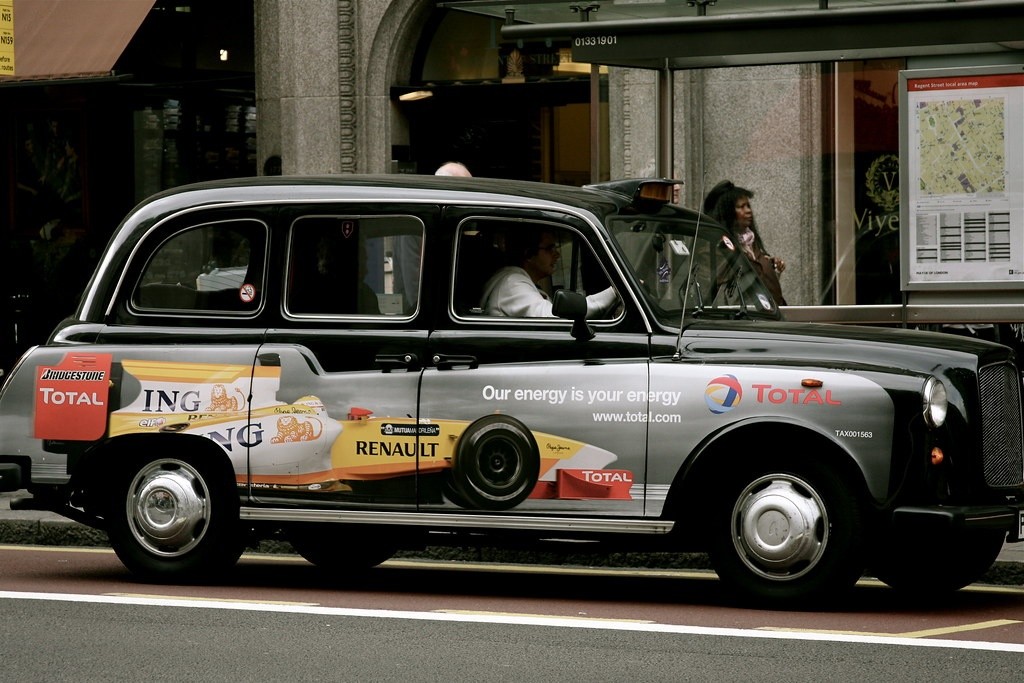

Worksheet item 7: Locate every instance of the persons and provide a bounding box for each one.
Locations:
[57,138,81,198]
[479,230,619,319]
[672,181,788,306]
[393,161,473,314]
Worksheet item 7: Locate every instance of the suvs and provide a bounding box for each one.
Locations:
[0,159,1023,607]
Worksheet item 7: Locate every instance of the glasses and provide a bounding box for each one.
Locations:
[539,243,557,253]
